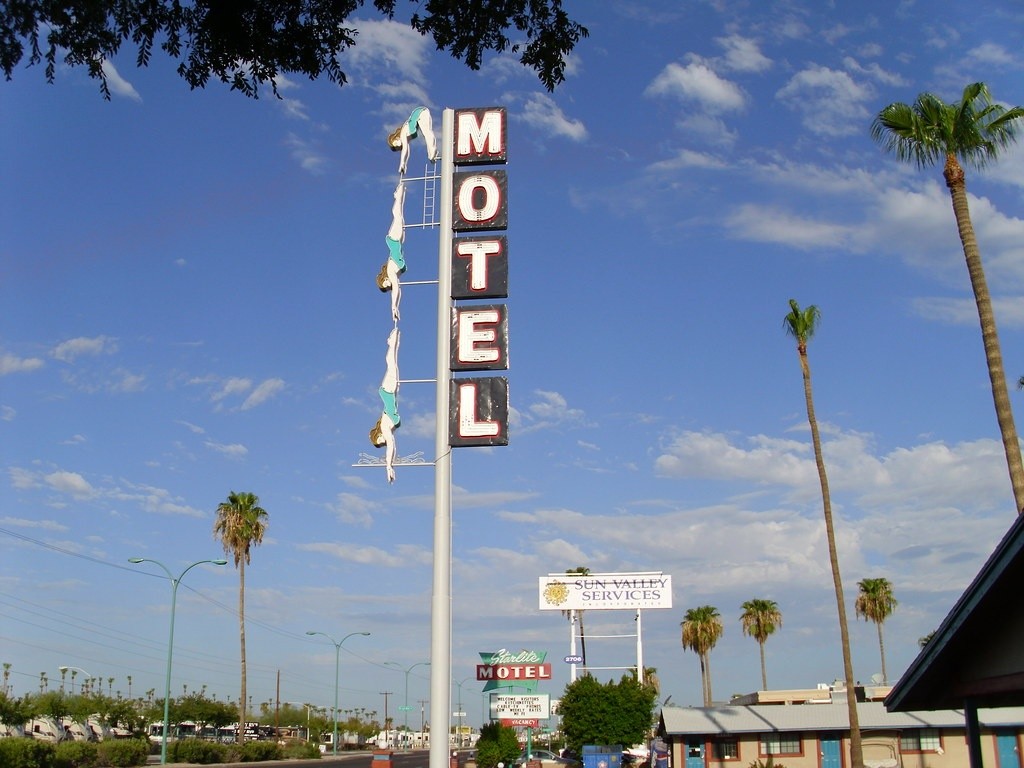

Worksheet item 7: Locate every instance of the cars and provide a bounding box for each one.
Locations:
[512,748,583,768]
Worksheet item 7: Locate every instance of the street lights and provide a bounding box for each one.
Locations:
[125,556,230,765]
[59,665,92,700]
[286,701,311,743]
[384,661,431,753]
[466,688,499,727]
[305,630,373,756]
